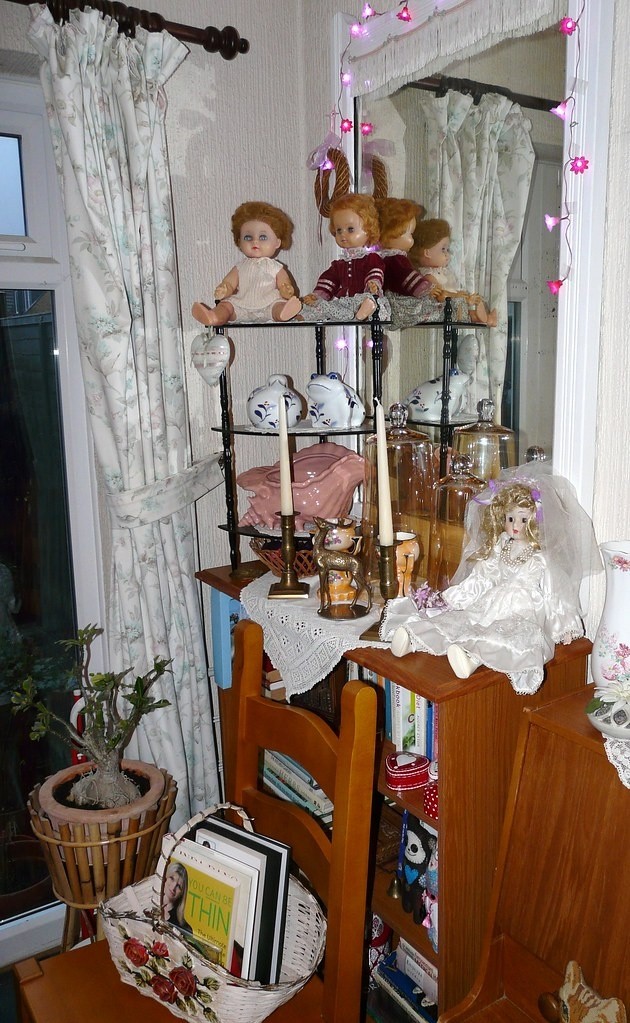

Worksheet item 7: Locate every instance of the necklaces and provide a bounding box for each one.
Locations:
[500,540,533,566]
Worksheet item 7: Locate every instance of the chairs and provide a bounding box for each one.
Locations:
[13,618,378,1023]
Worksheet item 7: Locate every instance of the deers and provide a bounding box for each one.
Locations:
[312,515,373,614]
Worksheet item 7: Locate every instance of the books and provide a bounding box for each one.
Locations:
[257,748,334,831]
[151,815,291,986]
[376,937,438,1023]
[260,653,287,703]
[347,660,439,763]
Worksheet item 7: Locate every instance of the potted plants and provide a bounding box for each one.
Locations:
[10,621,177,866]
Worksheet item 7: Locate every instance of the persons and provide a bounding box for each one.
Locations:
[162,862,194,934]
[374,198,470,331]
[191,202,302,327]
[381,460,604,696]
[410,219,498,326]
[298,195,392,330]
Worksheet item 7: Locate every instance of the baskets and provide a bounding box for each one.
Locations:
[98,802,328,1023]
[248,538,318,579]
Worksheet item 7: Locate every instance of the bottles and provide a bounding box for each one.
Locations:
[452,399,516,480]
[428,453,487,593]
[591,540,630,689]
[363,403,438,606]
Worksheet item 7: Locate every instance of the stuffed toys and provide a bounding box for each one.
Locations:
[401,814,439,954]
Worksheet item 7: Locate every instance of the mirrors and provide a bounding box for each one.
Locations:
[330,0,618,534]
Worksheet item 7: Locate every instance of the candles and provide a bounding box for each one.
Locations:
[277,394,294,516]
[373,396,395,547]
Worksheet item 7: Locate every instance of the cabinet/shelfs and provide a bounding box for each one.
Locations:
[195,558,595,1023]
